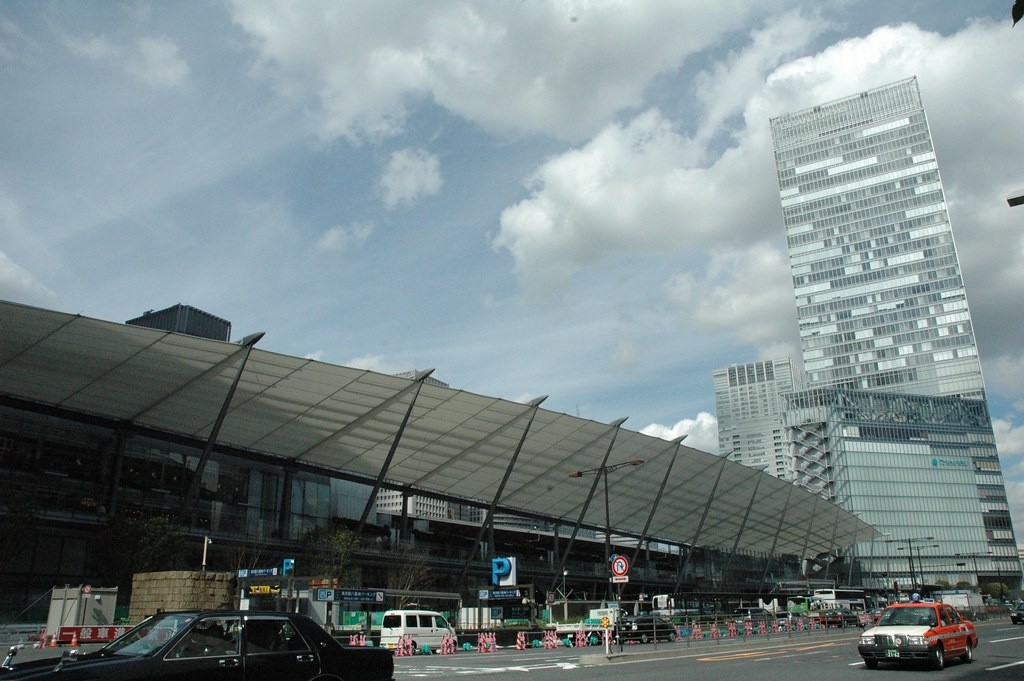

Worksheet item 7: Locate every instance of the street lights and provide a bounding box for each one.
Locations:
[955,551,993,594]
[885,537,939,595]
[569,460,643,598]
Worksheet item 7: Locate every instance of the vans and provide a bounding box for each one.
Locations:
[732,607,774,621]
[380,610,458,654]
[776,611,809,625]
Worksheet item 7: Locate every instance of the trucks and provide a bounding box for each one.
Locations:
[546,608,622,646]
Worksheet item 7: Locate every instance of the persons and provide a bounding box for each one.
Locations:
[204,624,226,656]
[818,600,823,605]
[811,600,816,606]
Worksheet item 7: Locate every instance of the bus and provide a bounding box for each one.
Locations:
[814,588,866,618]
[652,592,779,622]
[787,596,814,622]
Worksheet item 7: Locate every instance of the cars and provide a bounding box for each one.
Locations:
[1010,603,1024,625]
[874,607,893,620]
[0,610,394,681]
[612,615,677,644]
[819,609,860,628]
[983,601,1009,614]
[858,600,978,670]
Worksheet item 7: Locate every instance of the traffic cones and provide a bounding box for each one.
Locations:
[69,631,78,647]
[49,632,58,647]
